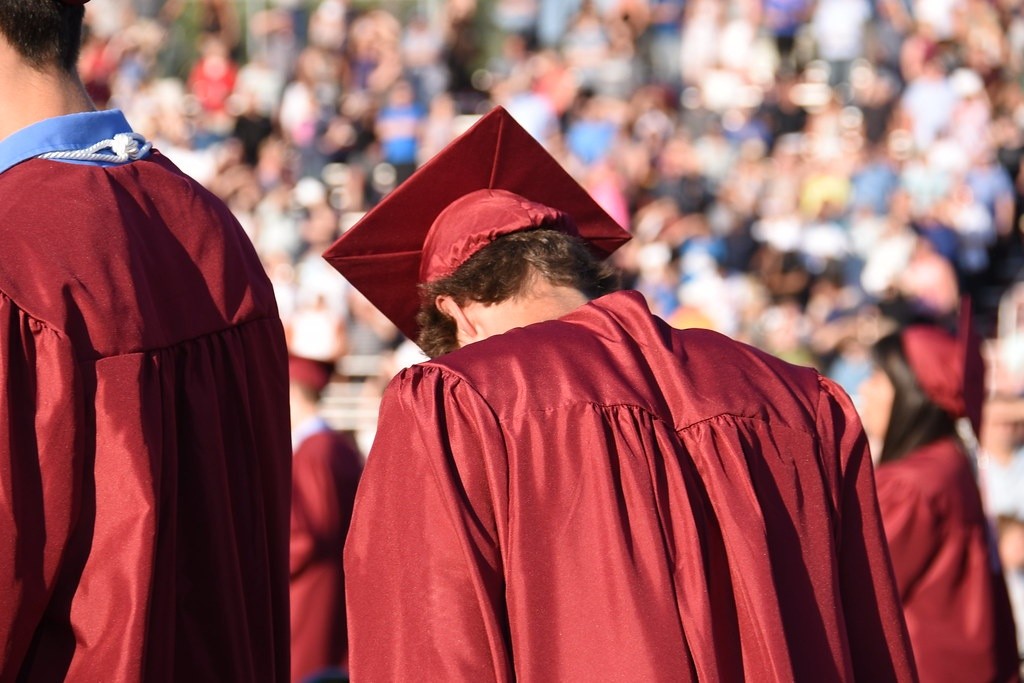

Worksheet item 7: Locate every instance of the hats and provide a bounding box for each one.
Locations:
[901,296,985,446]
[290,351,334,393]
[321,105,634,359]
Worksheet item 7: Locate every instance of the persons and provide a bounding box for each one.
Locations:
[2,1,1024,682]
[323,105,919,683]
